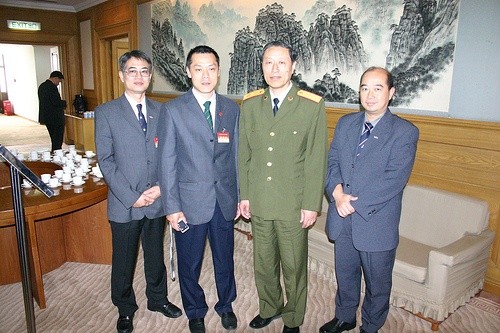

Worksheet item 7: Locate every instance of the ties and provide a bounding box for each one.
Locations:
[356,122,373,157]
[136,104,147,135]
[203,101,213,133]
[273,98,279,117]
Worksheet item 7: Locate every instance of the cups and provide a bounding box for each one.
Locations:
[40,150,103,187]
[21,178,33,189]
[83,111,94,119]
[10,150,40,161]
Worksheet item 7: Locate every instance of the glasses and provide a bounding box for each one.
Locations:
[124,68,152,77]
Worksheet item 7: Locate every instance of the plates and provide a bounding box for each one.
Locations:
[59,179,72,183]
[46,183,62,188]
[69,182,85,186]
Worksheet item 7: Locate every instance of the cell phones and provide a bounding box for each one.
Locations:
[177,218,190,233]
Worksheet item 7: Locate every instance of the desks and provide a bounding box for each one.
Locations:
[65,112,94,154]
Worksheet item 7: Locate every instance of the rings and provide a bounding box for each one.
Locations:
[145,202,148,206]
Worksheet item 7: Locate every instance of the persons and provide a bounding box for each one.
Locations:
[94,50,183,333]
[157,45,241,333]
[237,41,329,333]
[38,71,68,152]
[319,66,420,333]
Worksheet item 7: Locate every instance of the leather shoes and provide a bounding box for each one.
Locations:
[249,312,281,329]
[147,299,182,318]
[319,313,357,333]
[189,317,205,333]
[282,324,300,333]
[359,326,378,333]
[117,312,134,333]
[214,305,237,330]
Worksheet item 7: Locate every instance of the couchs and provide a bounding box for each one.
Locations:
[235,184,494,332]
